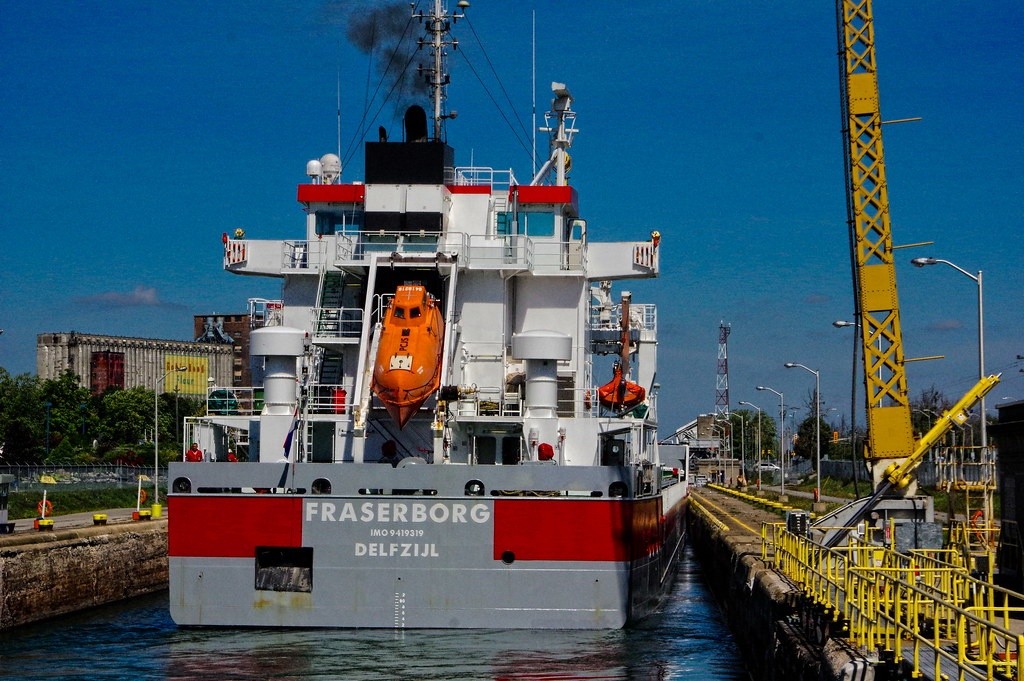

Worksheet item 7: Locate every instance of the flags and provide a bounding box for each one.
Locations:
[283,407,298,459]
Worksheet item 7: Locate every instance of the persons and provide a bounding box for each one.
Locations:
[185,442,202,463]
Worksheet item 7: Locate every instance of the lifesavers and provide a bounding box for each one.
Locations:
[140,489,146,503]
[813,487,819,502]
[756,478,759,486]
[970,509,994,547]
[37,500,52,515]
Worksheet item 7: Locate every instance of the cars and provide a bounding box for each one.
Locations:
[754,463,781,474]
[688,473,708,487]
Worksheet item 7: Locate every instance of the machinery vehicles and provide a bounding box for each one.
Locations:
[941,510,995,552]
[799,2,946,567]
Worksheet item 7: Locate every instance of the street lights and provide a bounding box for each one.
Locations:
[725,412,745,486]
[834,318,883,409]
[80,401,87,434]
[740,401,761,491]
[701,419,734,487]
[911,253,986,486]
[786,361,821,502]
[152,365,188,517]
[45,402,53,455]
[756,385,784,495]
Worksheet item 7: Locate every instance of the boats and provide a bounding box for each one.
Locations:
[162,0,693,629]
